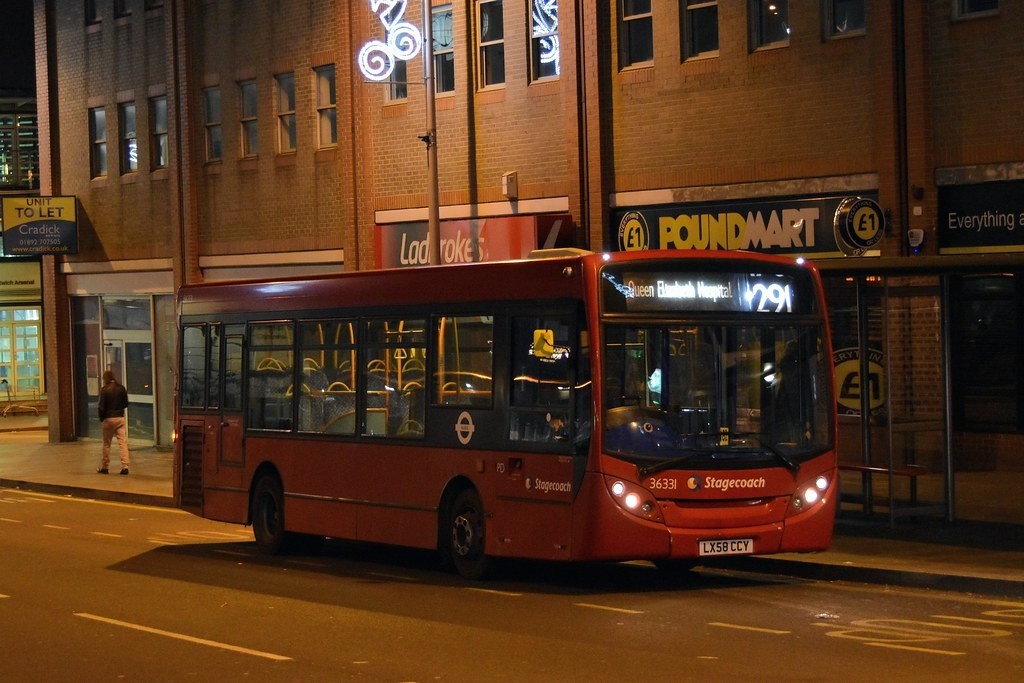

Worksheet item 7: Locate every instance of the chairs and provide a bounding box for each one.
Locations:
[258,357,465,435]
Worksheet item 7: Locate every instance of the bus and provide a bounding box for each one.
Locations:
[169,246,840,577]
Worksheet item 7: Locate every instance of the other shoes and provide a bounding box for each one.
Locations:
[120,469,129,474]
[97,467,108,474]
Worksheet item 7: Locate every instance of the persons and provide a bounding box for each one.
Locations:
[96,371,129,474]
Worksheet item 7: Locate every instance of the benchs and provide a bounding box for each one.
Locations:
[838,461,930,516]
